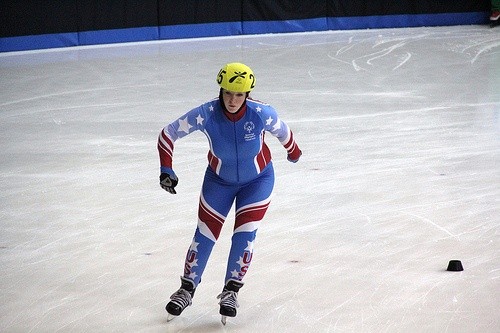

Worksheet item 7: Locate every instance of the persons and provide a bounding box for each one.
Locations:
[149,62,303,324]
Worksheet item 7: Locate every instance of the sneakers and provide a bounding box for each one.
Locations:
[219,278,243,326]
[164,276,201,323]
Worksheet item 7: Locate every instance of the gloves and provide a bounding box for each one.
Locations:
[287,142,302,163]
[160,173,178,194]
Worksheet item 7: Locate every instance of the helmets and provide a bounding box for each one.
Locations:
[217,62,256,93]
